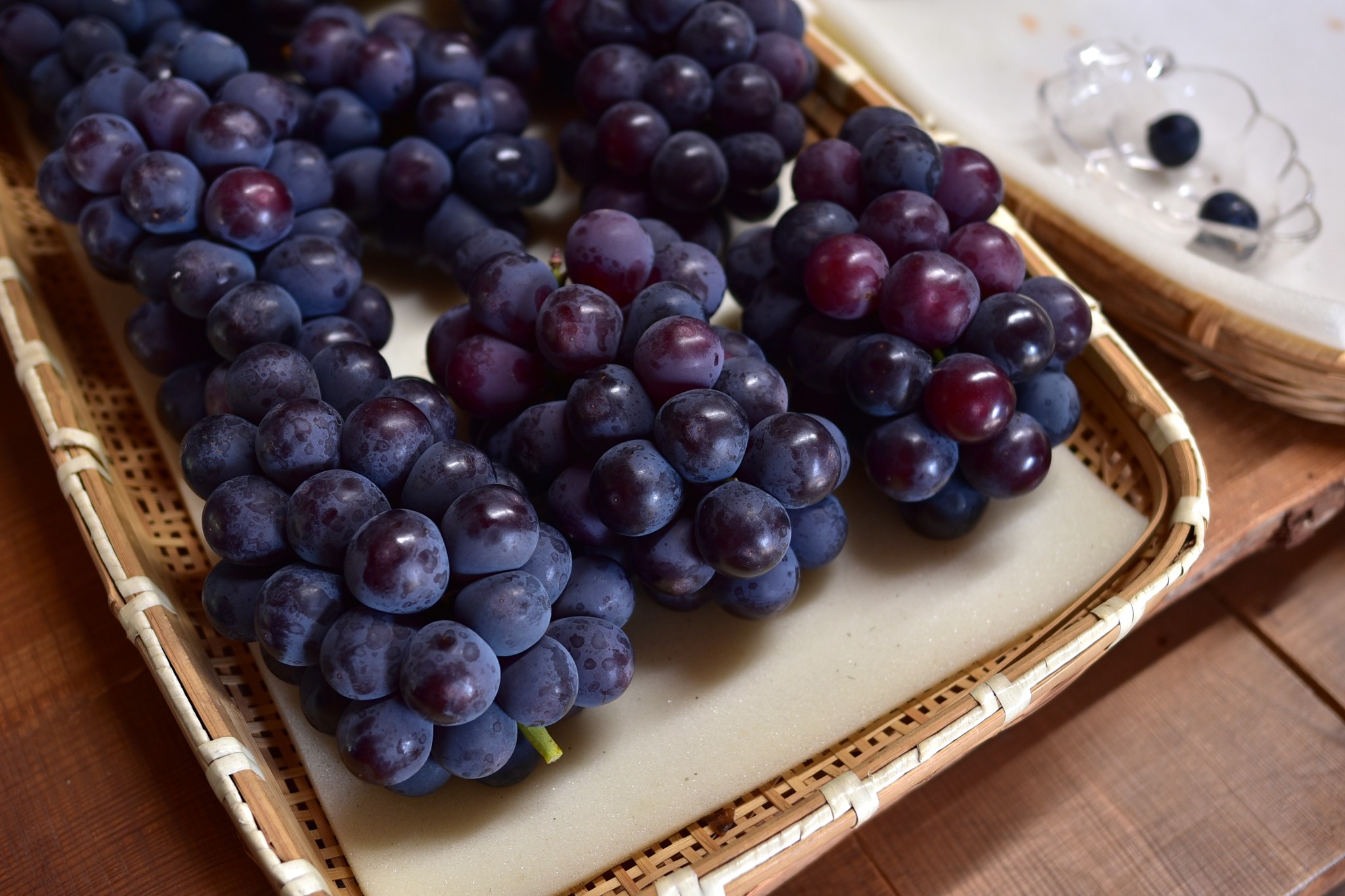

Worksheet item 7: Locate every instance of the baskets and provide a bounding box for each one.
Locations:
[0,1,1212,895]
[799,0,1345,427]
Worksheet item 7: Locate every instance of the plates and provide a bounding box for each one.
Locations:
[809,0,1345,352]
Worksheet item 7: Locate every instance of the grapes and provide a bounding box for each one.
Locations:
[1147,114,1199,168]
[1199,192,1260,231]
[0,0,1093,798]
